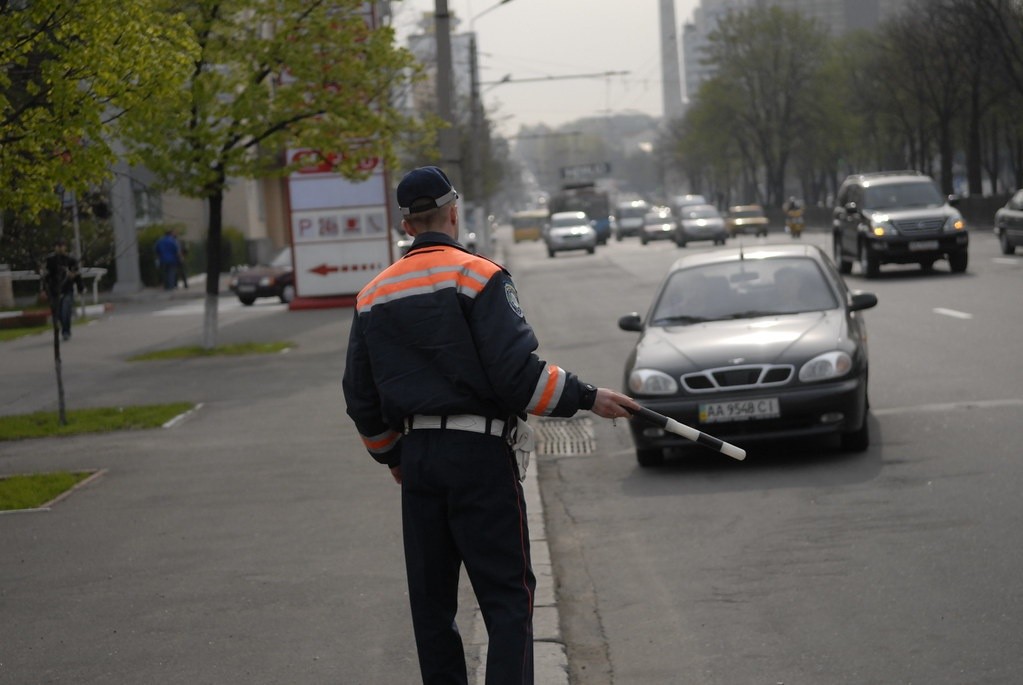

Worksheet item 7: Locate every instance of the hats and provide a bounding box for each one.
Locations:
[397,166,458,215]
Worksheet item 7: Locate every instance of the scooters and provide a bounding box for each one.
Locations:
[786,209,805,239]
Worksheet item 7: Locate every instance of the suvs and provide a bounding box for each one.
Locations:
[831,169,970,280]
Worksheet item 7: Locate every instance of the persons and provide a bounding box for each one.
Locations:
[788,197,800,210]
[341,166,641,685]
[154,229,190,290]
[775,267,802,302]
[42,239,86,340]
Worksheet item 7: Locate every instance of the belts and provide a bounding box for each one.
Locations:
[404,414,505,437]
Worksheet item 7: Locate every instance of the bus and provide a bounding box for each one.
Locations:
[509,210,541,245]
[546,186,611,245]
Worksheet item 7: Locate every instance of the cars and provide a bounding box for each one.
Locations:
[994,188,1023,255]
[618,241,877,469]
[641,210,678,246]
[674,204,728,247]
[728,203,769,239]
[543,211,599,259]
[228,244,297,307]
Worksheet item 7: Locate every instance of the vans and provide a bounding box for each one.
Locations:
[609,193,707,243]
